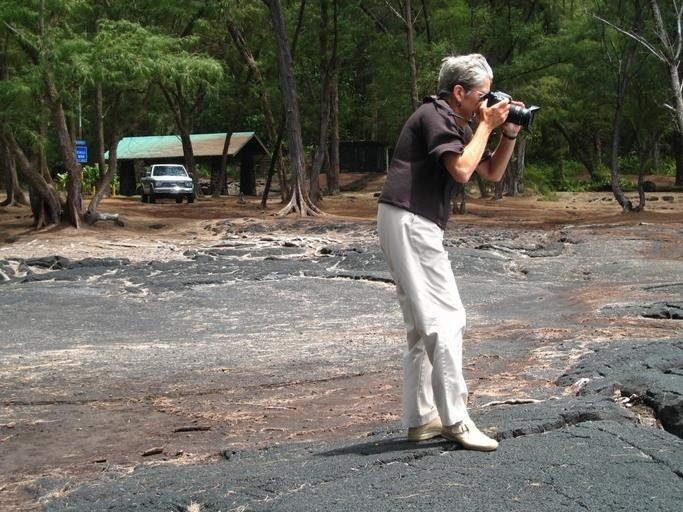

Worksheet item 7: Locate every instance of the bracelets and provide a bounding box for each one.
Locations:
[502,131,518,139]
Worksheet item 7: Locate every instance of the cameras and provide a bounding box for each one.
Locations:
[481,91,540,134]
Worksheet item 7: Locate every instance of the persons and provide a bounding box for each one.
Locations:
[377,52,526,452]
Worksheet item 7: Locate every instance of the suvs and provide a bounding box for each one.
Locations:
[140,164,196,205]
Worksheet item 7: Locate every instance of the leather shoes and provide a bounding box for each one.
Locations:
[440,419,498,451]
[408,415,440,441]
